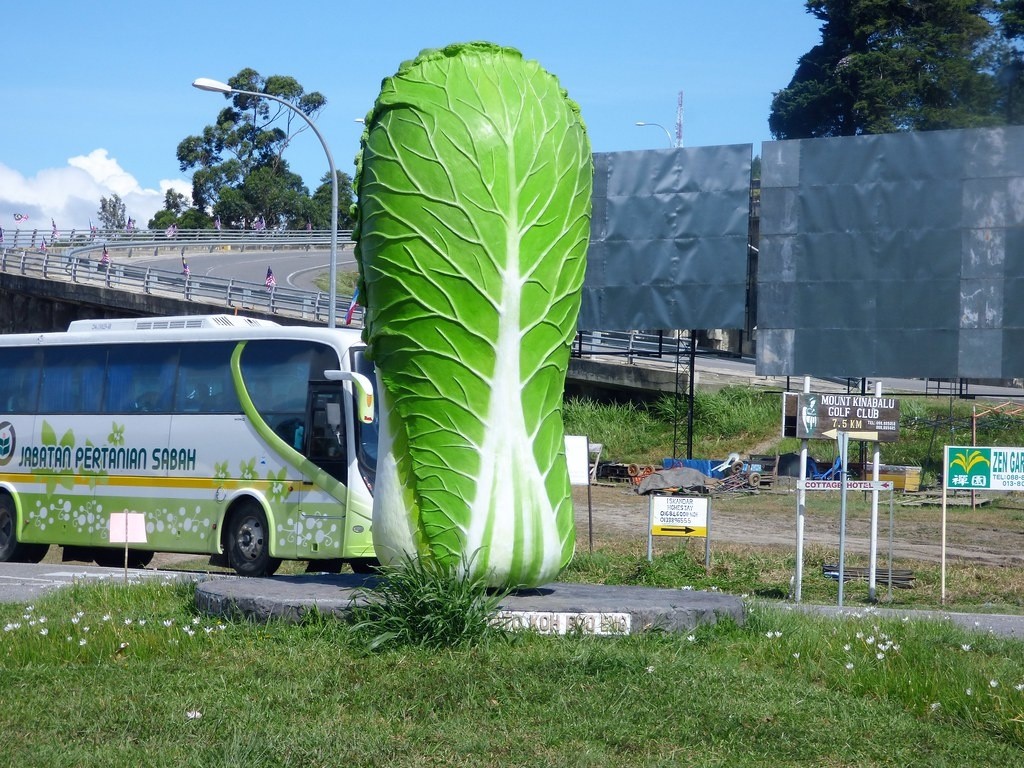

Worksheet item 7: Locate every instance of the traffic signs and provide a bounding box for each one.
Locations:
[650,496,707,538]
[796,392,902,444]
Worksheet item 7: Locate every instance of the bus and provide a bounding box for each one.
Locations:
[0,314,380,577]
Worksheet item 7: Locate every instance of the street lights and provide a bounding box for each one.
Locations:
[194,78,339,328]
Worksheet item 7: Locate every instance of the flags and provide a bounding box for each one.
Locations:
[0,222,3,242]
[264,266,279,294]
[13,213,29,224]
[180,253,190,278]
[214,215,313,231]
[344,285,360,325]
[35,216,179,271]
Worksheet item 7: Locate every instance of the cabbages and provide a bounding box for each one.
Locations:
[348,40,596,587]
